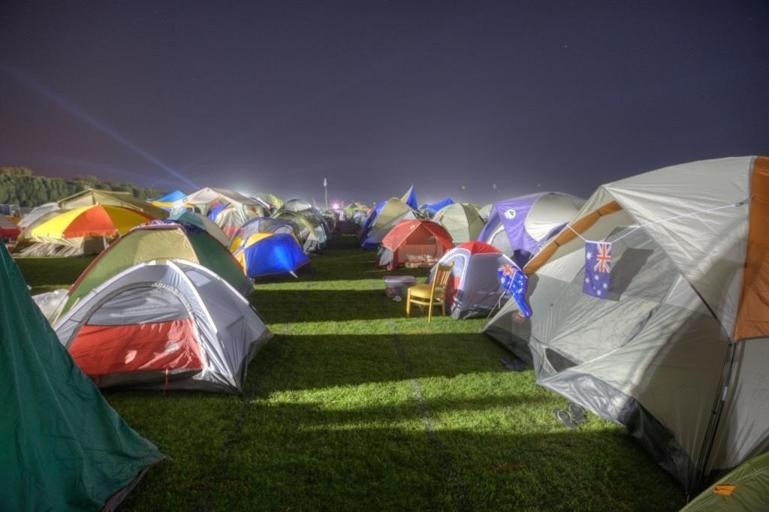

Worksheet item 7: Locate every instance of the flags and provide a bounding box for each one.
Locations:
[582,238,614,302]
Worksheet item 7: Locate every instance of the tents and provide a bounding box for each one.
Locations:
[3,188,586,326]
[477,149,769,497]
[51,256,275,402]
[0,241,174,512]
[672,446,769,509]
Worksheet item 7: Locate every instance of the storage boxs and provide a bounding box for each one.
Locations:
[384,274,416,301]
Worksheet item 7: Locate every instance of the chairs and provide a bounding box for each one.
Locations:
[406,263,454,323]
